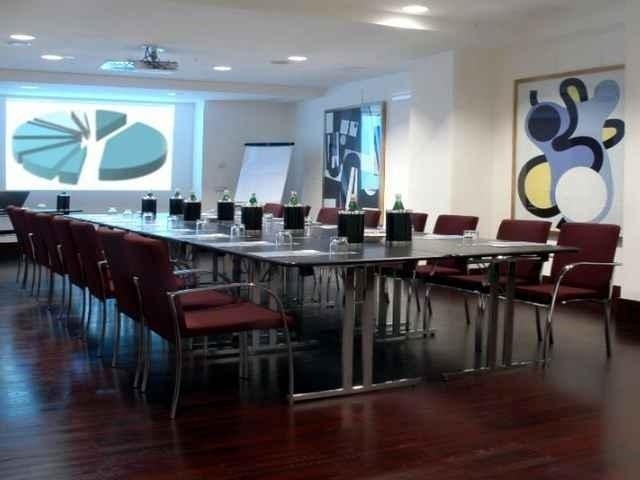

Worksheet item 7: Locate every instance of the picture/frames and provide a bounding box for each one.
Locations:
[321,100,385,226]
[511,62,623,250]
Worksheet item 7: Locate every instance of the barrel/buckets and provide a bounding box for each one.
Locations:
[283,204,304,236]
[337,209,368,248]
[184,202,201,225]
[142,198,156,215]
[57,195,70,212]
[241,206,263,235]
[168,198,183,217]
[217,201,235,225]
[386,209,413,246]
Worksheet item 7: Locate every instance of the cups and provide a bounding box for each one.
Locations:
[57,192,71,215]
[263,210,273,223]
[183,201,202,221]
[336,208,365,243]
[384,210,412,241]
[231,223,245,241]
[193,219,207,236]
[141,197,157,219]
[272,229,293,250]
[241,205,265,231]
[167,197,184,216]
[329,234,351,256]
[216,200,233,221]
[283,203,305,230]
[108,208,184,229]
[461,230,479,245]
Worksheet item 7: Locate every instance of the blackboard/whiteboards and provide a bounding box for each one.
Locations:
[233,143,294,207]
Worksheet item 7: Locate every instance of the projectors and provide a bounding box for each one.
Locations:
[134,48,177,71]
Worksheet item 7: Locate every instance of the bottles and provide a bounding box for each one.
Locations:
[288,191,299,204]
[221,188,232,201]
[248,191,258,206]
[144,187,199,200]
[391,193,405,210]
[348,196,360,210]
[61,188,68,195]
